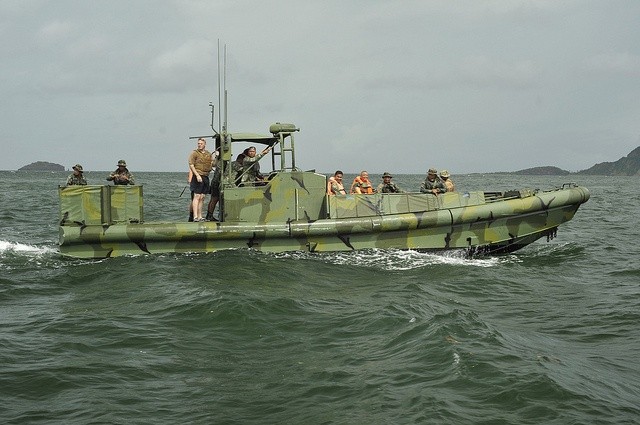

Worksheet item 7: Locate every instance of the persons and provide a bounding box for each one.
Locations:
[65,164,88,185]
[326,170,347,197]
[234,149,248,186]
[188,166,195,222]
[188,138,220,222]
[439,169,455,192]
[418,167,448,197]
[105,160,136,185]
[376,172,400,193]
[241,146,268,186]
[205,146,232,222]
[349,170,375,196]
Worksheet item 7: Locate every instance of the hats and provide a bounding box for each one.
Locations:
[427,168,438,175]
[72,164,84,174]
[382,173,392,178]
[440,170,449,178]
[117,160,127,166]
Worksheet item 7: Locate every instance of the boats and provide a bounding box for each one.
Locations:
[57,36,590,271]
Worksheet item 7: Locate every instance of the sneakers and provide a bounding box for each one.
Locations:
[193,218,199,222]
[200,218,209,222]
[207,215,218,221]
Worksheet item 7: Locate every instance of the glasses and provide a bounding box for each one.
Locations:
[250,147,255,148]
[198,138,204,141]
